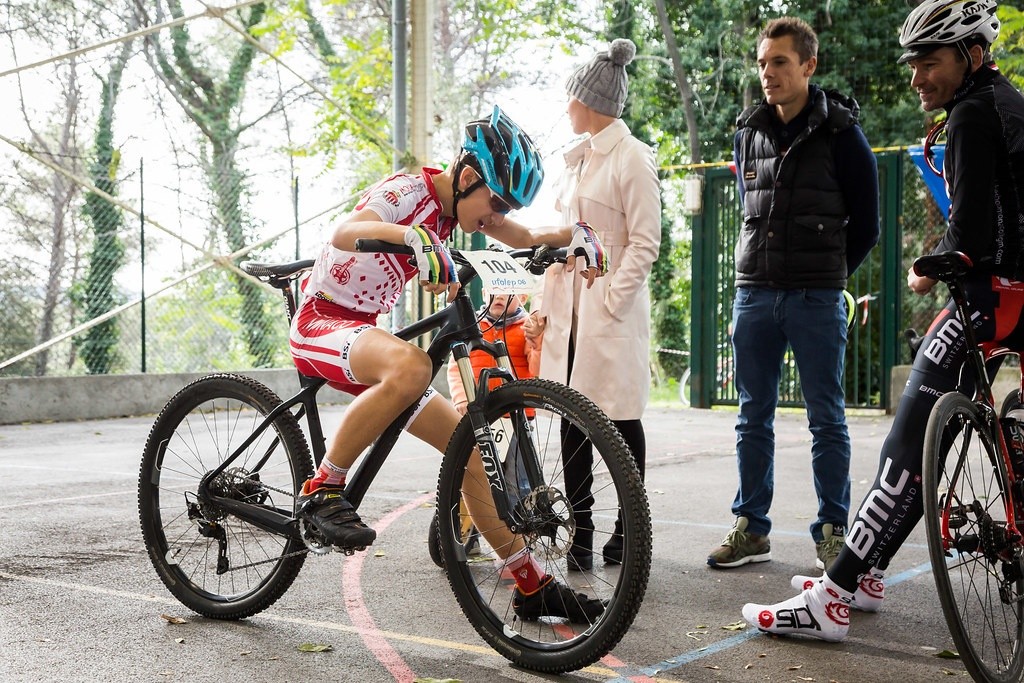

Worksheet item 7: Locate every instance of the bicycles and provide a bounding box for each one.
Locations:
[913,249,1024,683]
[137,238,651,673]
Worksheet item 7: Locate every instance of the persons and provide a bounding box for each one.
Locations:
[703,20,881,571]
[288,102,621,627]
[743,0,1023,644]
[445,268,551,555]
[525,55,664,572]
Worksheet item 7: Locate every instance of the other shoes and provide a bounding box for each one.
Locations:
[568,517,595,570]
[466,540,479,554]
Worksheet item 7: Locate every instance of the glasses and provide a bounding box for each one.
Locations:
[464,162,512,214]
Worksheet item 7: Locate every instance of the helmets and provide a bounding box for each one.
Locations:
[460,105,545,211]
[897,0,999,66]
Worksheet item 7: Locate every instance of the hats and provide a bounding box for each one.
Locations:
[565,37,636,118]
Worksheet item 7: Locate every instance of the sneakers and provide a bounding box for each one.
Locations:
[296,479,377,547]
[789,571,887,612]
[742,591,853,643]
[811,526,850,573]
[602,522,627,564]
[707,516,774,569]
[512,574,609,623]
